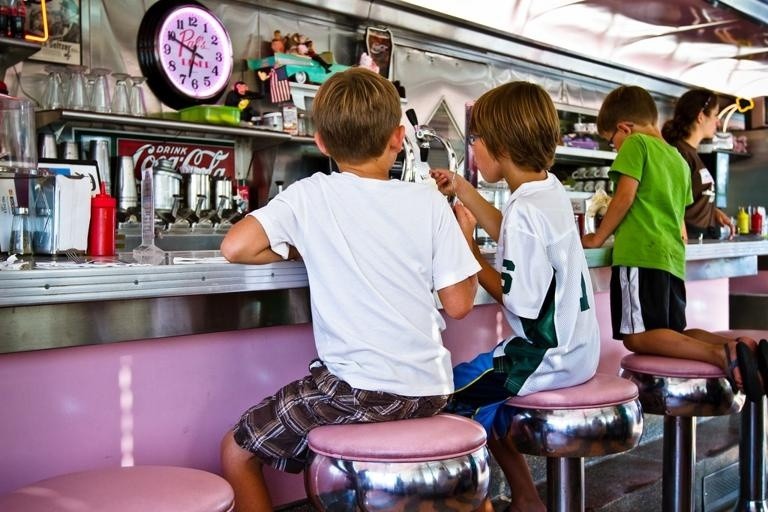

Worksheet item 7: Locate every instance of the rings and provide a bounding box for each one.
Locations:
[728,222,733,226]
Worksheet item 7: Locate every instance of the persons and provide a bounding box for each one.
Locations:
[660,88,735,243]
[430,78,602,512]
[218,67,486,511]
[579,85,768,401]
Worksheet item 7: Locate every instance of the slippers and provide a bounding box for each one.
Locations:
[735,337,768,396]
[722,342,762,404]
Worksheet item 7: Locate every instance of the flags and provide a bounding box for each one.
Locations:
[270,66,290,103]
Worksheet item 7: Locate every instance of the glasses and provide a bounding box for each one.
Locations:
[608,122,633,150]
[467,132,488,146]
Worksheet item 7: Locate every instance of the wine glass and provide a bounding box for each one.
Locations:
[43,63,148,116]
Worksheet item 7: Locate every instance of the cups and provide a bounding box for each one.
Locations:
[573,122,598,134]
[571,165,614,194]
[40,133,139,211]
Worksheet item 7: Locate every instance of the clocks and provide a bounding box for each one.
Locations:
[132,1,238,109]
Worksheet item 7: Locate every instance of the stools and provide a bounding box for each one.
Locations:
[0,462,236,512]
[495,368,648,511]
[615,344,746,512]
[303,408,492,511]
[711,324,768,512]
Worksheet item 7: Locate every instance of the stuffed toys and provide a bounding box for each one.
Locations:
[272,31,332,73]
[225,70,270,126]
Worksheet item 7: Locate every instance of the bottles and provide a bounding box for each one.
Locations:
[11,206,54,254]
[251,106,306,138]
[86,181,116,257]
[0,0,27,40]
[737,205,762,235]
